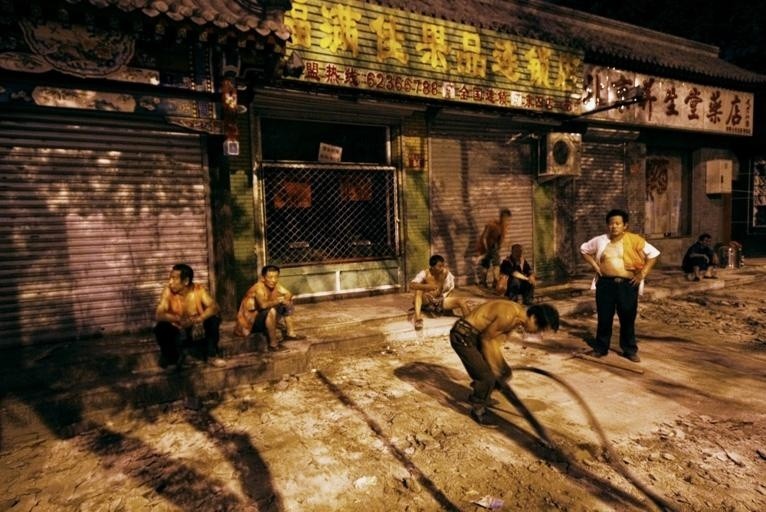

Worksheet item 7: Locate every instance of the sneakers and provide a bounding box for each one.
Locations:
[466,393,502,430]
[620,350,640,363]
[204,356,227,368]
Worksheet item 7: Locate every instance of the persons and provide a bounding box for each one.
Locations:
[474,206,512,286]
[151,263,227,372]
[232,263,306,352]
[450,297,561,430]
[407,253,470,331]
[681,233,720,282]
[496,243,538,305]
[579,207,661,362]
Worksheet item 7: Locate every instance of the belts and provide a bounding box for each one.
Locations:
[601,275,630,284]
[455,318,481,338]
